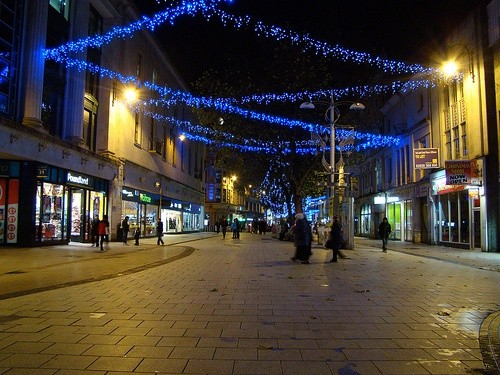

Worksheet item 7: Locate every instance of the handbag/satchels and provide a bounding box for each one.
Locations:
[325,239,334,249]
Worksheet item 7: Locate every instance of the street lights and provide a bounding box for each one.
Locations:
[300,94,366,250]
[262,188,268,223]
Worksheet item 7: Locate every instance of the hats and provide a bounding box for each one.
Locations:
[295,213,303,219]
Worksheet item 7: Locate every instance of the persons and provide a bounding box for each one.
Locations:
[313,220,322,245]
[377,217,392,253]
[169,218,176,229]
[291,213,312,264]
[330,216,346,262]
[252,217,267,235]
[231,218,240,238]
[134,227,141,246]
[122,216,129,245]
[215,217,228,240]
[156,218,164,245]
[91,215,109,251]
[279,222,296,241]
